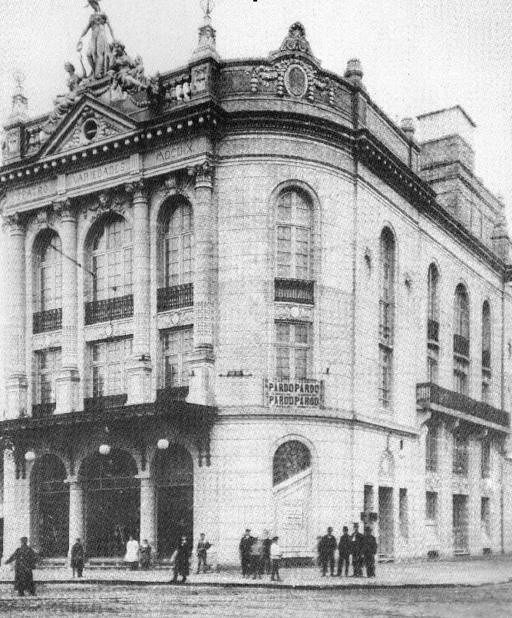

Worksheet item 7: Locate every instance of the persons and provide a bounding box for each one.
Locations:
[171,534,190,583]
[6,537,39,595]
[124,536,151,570]
[240,528,283,582]
[78,1,116,77]
[71,538,84,577]
[316,523,377,578]
[196,533,209,574]
